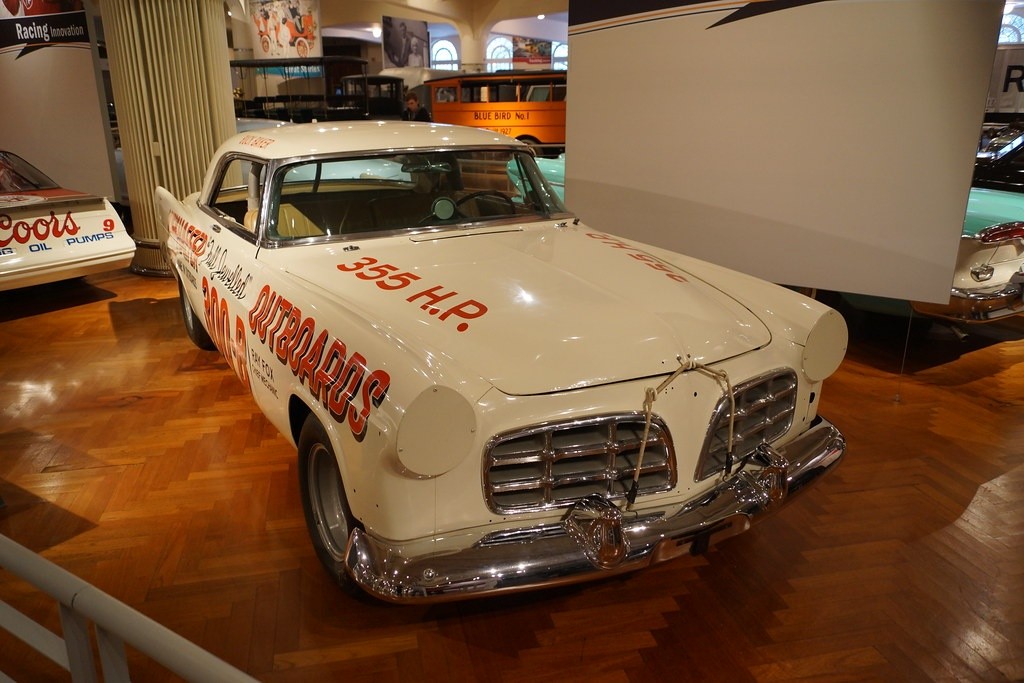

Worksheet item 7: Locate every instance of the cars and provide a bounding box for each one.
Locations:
[153,120,849,606]
[972,133,1024,184]
[0,149,137,293]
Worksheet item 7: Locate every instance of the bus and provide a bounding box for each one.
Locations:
[424,69,567,158]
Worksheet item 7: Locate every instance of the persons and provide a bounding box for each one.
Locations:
[337,81,347,96]
[409,38,423,66]
[397,23,411,67]
[404,92,431,121]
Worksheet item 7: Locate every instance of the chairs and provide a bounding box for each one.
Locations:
[364,199,432,235]
[277,199,362,239]
